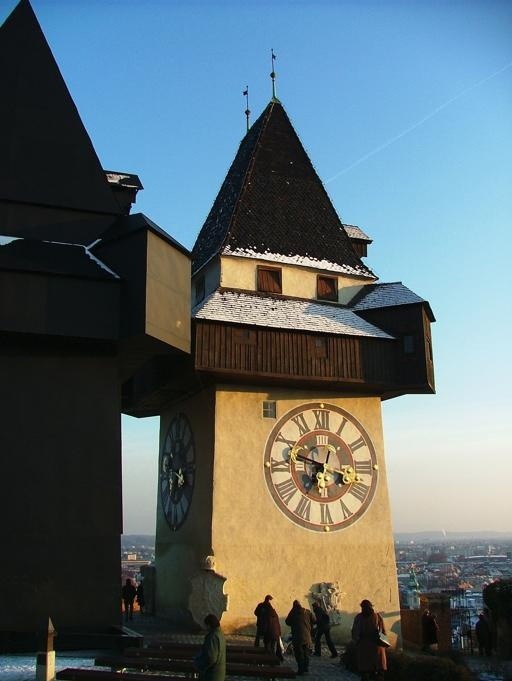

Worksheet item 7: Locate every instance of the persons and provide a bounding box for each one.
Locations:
[254,595,273,647]
[312,602,337,658]
[137,579,144,614]
[477,614,490,657]
[191,614,226,681]
[434,615,440,641]
[123,578,138,623]
[262,604,283,655]
[423,608,437,656]
[285,600,317,675]
[351,598,388,681]
[481,608,497,651]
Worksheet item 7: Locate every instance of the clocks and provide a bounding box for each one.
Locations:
[262,401,379,533]
[159,412,196,533]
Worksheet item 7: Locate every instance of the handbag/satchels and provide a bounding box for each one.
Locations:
[375,630,391,647]
